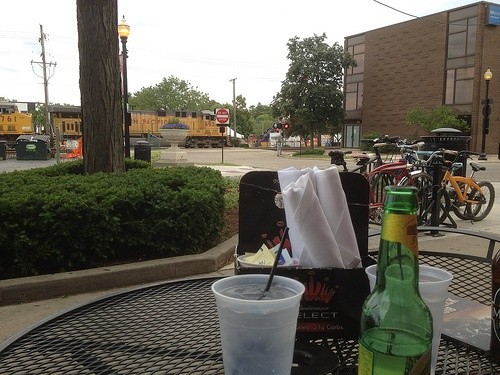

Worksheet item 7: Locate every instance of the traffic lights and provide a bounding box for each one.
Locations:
[284,123,290,129]
[273,122,278,128]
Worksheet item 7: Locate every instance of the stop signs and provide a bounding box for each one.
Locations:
[216,109,228,123]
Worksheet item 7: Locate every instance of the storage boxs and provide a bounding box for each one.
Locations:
[233,171,378,337]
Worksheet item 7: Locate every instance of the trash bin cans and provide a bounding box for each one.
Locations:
[15,134,50,161]
[421,128,471,210]
[0,141,8,160]
[135,141,152,162]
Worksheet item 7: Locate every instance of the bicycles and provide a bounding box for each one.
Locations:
[329,134,496,234]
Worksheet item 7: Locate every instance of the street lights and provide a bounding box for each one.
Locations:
[479,67,493,161]
[117,14,130,158]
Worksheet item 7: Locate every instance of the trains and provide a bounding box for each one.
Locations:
[0,100,248,161]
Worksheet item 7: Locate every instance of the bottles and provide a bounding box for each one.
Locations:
[490,248,500,364]
[356,185,433,375]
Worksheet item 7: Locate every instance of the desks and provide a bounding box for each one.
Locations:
[0,250,500,375]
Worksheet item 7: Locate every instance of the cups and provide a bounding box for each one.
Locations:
[236,254,295,267]
[210,273,306,375]
[365,264,454,375]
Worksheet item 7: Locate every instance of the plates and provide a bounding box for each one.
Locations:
[291,340,339,375]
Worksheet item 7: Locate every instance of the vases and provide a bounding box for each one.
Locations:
[160,129,189,152]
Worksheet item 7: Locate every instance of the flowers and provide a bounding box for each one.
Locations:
[159,118,189,130]
